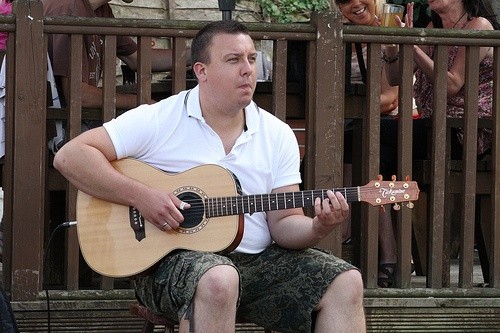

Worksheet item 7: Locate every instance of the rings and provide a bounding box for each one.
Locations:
[162,222,168,227]
[180,201,187,210]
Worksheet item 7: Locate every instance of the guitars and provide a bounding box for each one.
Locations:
[74,157,420,279]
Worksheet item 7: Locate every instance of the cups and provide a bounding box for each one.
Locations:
[382,4,405,47]
[261,40,273,81]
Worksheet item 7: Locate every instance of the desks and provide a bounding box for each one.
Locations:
[98,82,395,271]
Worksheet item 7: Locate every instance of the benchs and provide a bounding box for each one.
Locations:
[45,108,126,285]
[411,117,493,289]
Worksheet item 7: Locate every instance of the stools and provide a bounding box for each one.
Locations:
[130,303,281,333]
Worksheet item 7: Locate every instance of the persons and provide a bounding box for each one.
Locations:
[380,0,500,287]
[42,0,193,131]
[335,0,418,244]
[53,20,366,333]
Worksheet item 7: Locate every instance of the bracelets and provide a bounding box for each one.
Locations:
[383,52,399,64]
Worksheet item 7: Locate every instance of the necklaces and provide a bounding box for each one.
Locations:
[452,11,467,29]
